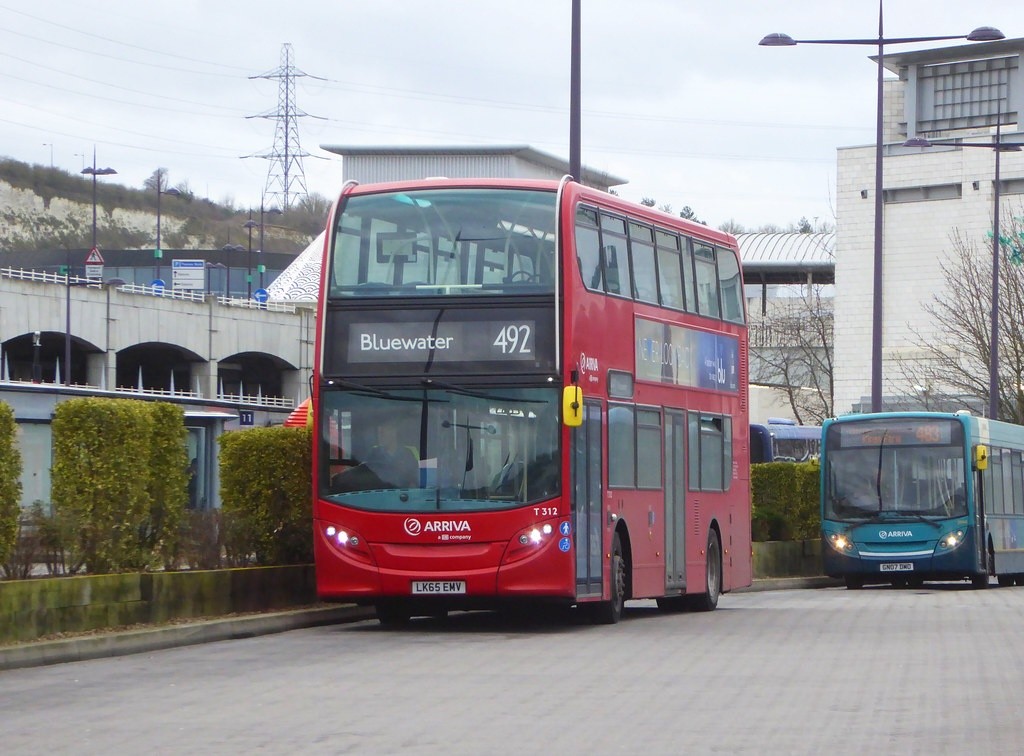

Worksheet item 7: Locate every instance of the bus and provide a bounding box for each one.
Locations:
[820,411,1024,586]
[751,418,823,467]
[309,180,752,624]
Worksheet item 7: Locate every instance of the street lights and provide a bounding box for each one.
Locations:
[906,138,1001,420]
[260,209,282,292]
[82,167,117,246]
[155,189,179,281]
[65,279,126,385]
[759,25,1004,411]
[224,243,246,298]
[243,222,263,294]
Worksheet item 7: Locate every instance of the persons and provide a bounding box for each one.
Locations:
[359,418,420,490]
